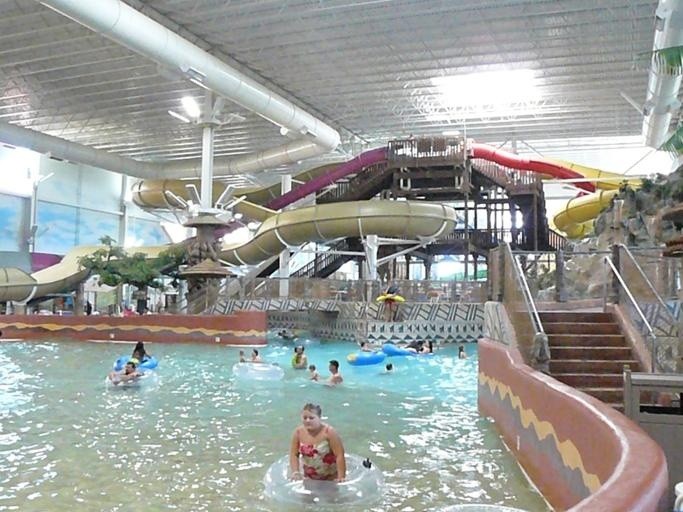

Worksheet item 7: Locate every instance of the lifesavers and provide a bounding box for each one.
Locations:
[233,361,285,381]
[439,503,524,511]
[264,450,383,504]
[346,349,384,365]
[382,344,414,355]
[106,356,158,389]
[377,293,405,302]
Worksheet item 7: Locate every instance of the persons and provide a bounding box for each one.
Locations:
[458,345,466,359]
[239,328,344,385]
[287,402,347,482]
[381,279,398,321]
[131,341,152,363]
[386,363,394,371]
[405,339,433,354]
[108,361,138,382]
[360,342,369,352]
